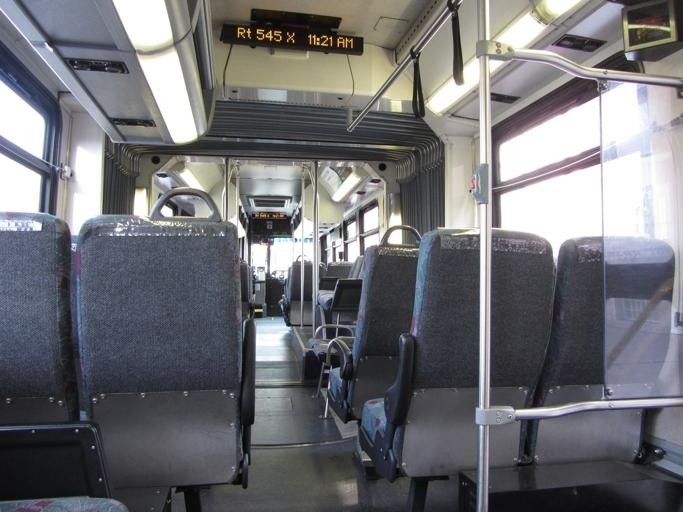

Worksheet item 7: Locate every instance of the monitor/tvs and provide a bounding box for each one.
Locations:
[251,218,292,238]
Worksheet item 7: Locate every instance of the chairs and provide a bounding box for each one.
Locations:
[0,187,257,512]
[282,225,683,512]
